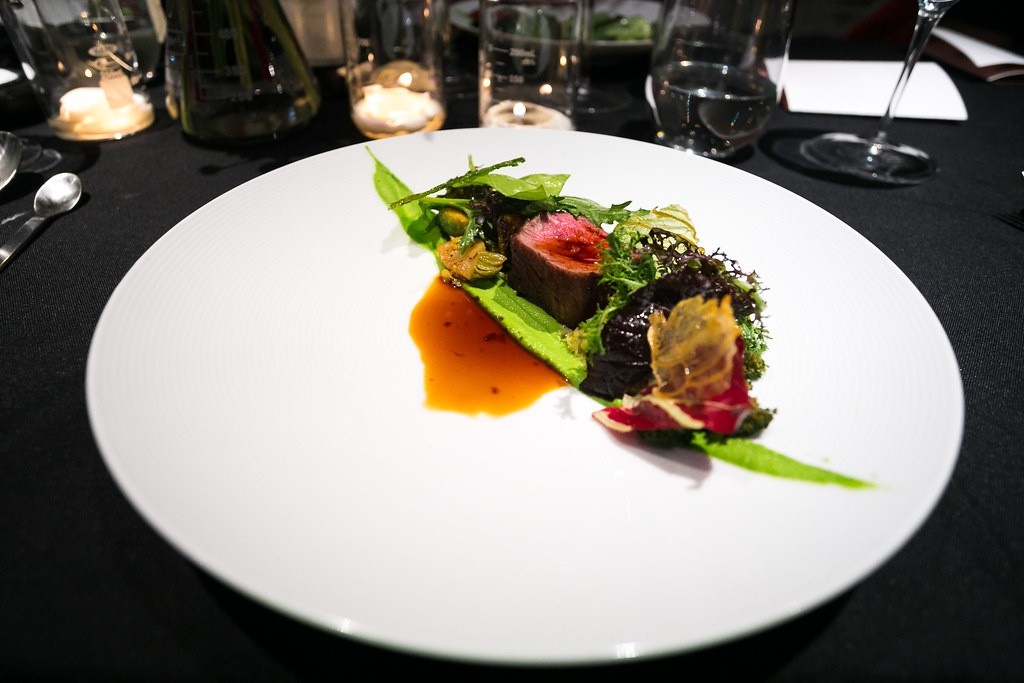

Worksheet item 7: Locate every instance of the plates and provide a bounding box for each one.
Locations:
[83,126,964,665]
[448,0,715,64]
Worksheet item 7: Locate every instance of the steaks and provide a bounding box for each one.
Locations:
[490,210,648,327]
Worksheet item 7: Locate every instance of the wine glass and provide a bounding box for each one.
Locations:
[799,0,957,185]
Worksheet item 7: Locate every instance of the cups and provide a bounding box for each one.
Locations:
[0,0,155,142]
[642,0,796,158]
[479,0,593,132]
[349,0,448,139]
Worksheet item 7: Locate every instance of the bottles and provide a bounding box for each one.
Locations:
[160,0,322,146]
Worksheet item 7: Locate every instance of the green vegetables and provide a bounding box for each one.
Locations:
[385,154,767,404]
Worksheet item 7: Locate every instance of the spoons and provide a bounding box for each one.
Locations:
[0,172,82,270]
[0,130,22,192]
